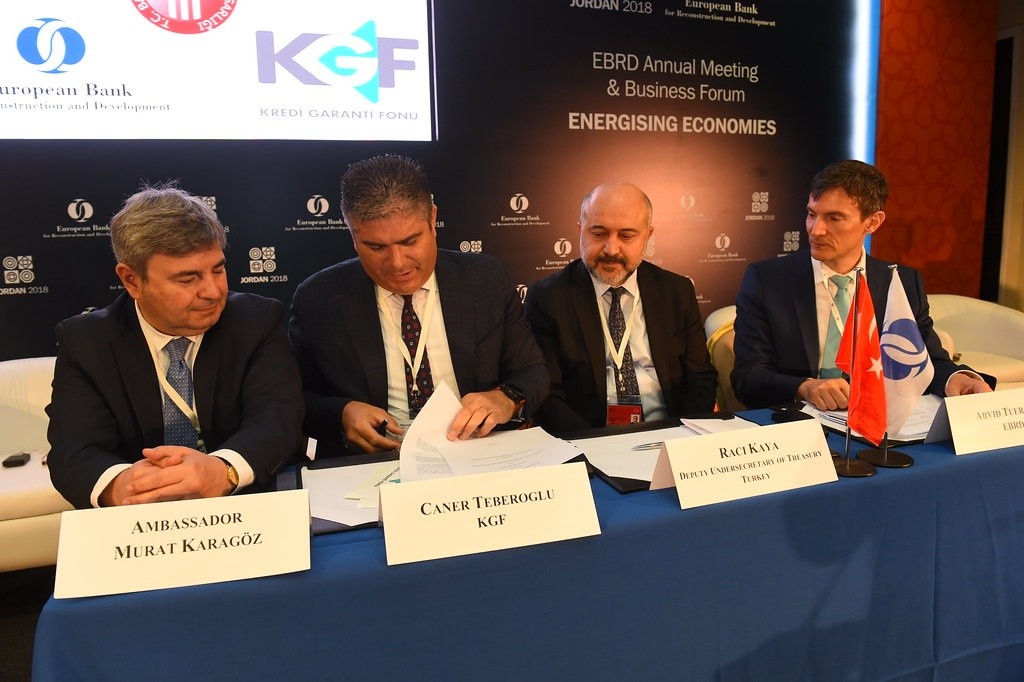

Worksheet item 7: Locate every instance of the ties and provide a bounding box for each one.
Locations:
[820,275,852,380]
[608,286,645,422]
[162,337,198,452]
[401,293,434,420]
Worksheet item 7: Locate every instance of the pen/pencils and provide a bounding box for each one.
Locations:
[632,441,665,452]
[376,419,389,434]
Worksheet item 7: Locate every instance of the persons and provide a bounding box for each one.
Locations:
[287,154,547,462]
[44,190,303,509]
[729,160,997,410]
[631,416,638,424]
[523,181,718,432]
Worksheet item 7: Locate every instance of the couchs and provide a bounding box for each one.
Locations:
[704,295,1024,410]
[0,357,77,575]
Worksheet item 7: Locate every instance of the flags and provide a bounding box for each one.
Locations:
[834,274,887,447]
[879,270,935,436]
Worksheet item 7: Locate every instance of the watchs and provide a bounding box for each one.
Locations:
[217,457,239,492]
[495,384,527,418]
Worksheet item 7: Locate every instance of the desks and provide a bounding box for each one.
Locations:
[30,403,1024,682]
